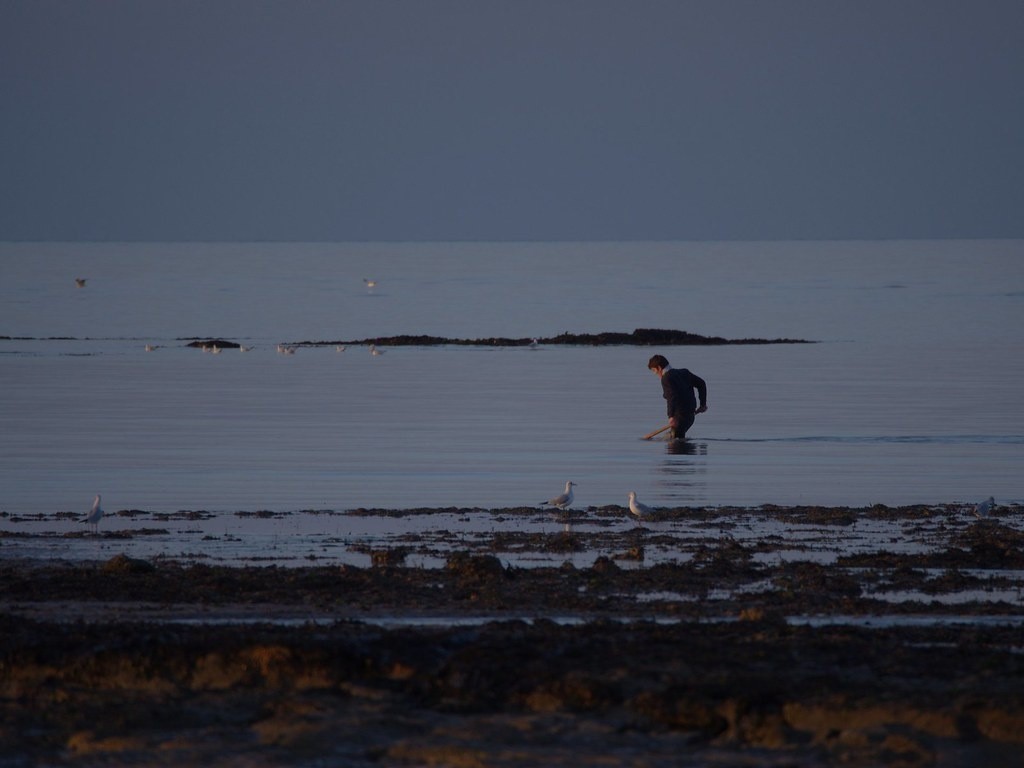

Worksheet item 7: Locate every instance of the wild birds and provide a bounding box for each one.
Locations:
[539,481,578,510]
[627,491,656,520]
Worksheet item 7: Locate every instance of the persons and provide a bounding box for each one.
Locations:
[648,355,707,439]
